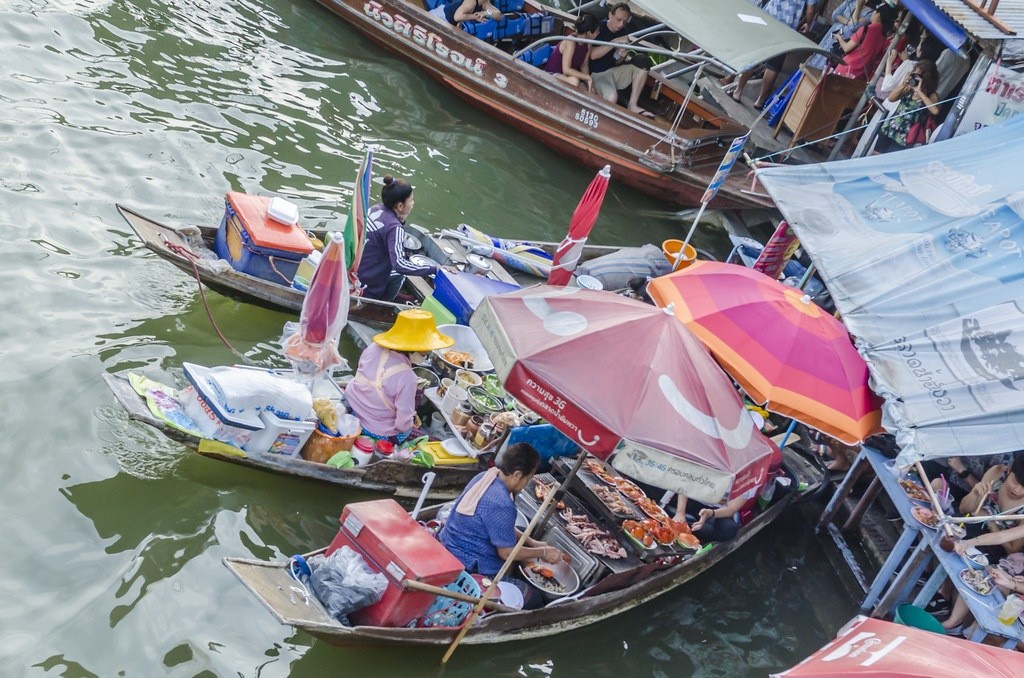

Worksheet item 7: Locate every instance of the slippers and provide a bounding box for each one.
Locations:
[635,107,655,119]
[812,444,835,459]
[825,461,850,473]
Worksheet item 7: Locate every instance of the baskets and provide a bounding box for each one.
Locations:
[303,421,364,463]
[423,571,482,626]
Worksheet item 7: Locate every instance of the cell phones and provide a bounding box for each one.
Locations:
[830,28,843,39]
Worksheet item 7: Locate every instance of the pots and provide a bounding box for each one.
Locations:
[451,254,492,277]
[404,232,421,252]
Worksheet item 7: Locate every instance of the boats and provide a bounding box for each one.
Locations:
[309,0,848,211]
[98,160,829,651]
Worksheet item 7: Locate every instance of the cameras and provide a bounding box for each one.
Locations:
[907,44,916,56]
[907,73,923,87]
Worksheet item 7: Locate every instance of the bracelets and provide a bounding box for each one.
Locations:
[1013,580,1018,590]
[711,510,715,518]
[542,547,545,559]
[959,469,969,478]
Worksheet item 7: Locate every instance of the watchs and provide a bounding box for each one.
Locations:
[486,9,492,18]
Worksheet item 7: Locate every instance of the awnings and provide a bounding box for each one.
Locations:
[740,108,1024,469]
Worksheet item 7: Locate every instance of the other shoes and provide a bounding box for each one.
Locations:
[883,505,965,636]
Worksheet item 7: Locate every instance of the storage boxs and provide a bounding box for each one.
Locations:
[432,268,522,327]
[182,362,266,448]
[324,497,465,629]
[234,364,317,459]
[213,193,315,287]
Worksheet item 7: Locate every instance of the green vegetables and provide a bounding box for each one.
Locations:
[471,392,496,408]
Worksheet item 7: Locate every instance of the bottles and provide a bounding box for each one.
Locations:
[369,440,393,464]
[350,437,375,467]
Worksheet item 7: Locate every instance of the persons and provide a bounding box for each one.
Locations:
[663,494,747,544]
[439,442,563,610]
[544,14,601,93]
[588,2,656,120]
[725,0,817,110]
[357,175,460,306]
[428,0,503,27]
[342,309,455,445]
[806,0,943,155]
[891,451,1024,652]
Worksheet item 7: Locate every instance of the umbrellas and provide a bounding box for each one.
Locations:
[767,614,1024,678]
[470,282,787,541]
[342,151,372,295]
[281,231,350,372]
[547,164,612,287]
[643,258,889,447]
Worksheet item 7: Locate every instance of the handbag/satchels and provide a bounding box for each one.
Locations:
[906,93,937,144]
[826,24,867,68]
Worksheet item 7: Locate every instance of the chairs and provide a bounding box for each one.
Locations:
[460,11,555,54]
[516,42,550,68]
[493,0,525,15]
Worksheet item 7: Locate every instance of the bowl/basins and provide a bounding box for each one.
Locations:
[518,556,580,599]
[960,546,989,571]
[433,324,494,371]
[454,370,536,435]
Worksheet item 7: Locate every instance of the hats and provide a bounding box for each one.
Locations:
[374,308,454,351]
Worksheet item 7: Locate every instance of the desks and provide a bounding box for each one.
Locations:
[773,63,876,156]
[814,445,951,612]
[868,526,1024,651]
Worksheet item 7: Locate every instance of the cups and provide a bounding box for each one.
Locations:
[441,385,468,416]
[996,592,1024,626]
[430,412,446,428]
[473,422,497,451]
[465,415,485,441]
[939,523,967,553]
[936,489,955,513]
[436,377,454,397]
[450,402,473,426]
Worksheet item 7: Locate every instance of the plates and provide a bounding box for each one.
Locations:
[593,470,617,486]
[676,536,701,549]
[910,506,942,530]
[958,568,995,596]
[637,497,669,524]
[614,477,647,501]
[898,481,933,503]
[624,528,658,551]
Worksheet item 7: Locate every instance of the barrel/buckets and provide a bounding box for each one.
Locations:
[412,367,441,406]
[662,238,697,271]
[893,604,946,635]
[576,275,603,291]
